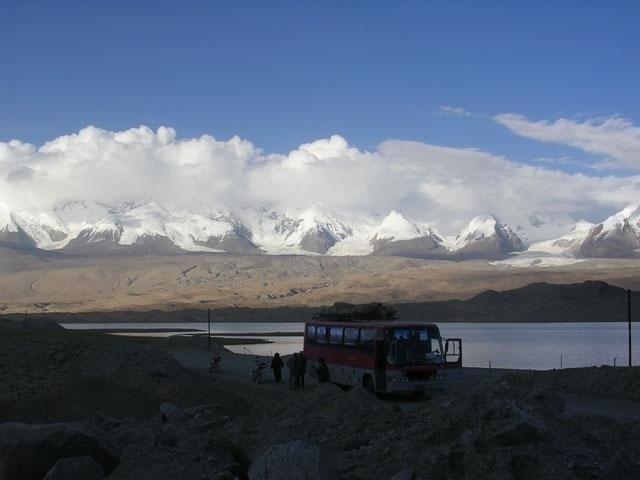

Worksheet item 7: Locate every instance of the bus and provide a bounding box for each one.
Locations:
[303,319,463,401]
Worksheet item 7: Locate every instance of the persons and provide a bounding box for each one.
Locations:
[311,356,331,383]
[270,352,285,384]
[296,349,308,389]
[287,351,305,392]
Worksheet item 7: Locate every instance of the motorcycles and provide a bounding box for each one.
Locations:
[250,355,271,384]
[208,352,222,374]
